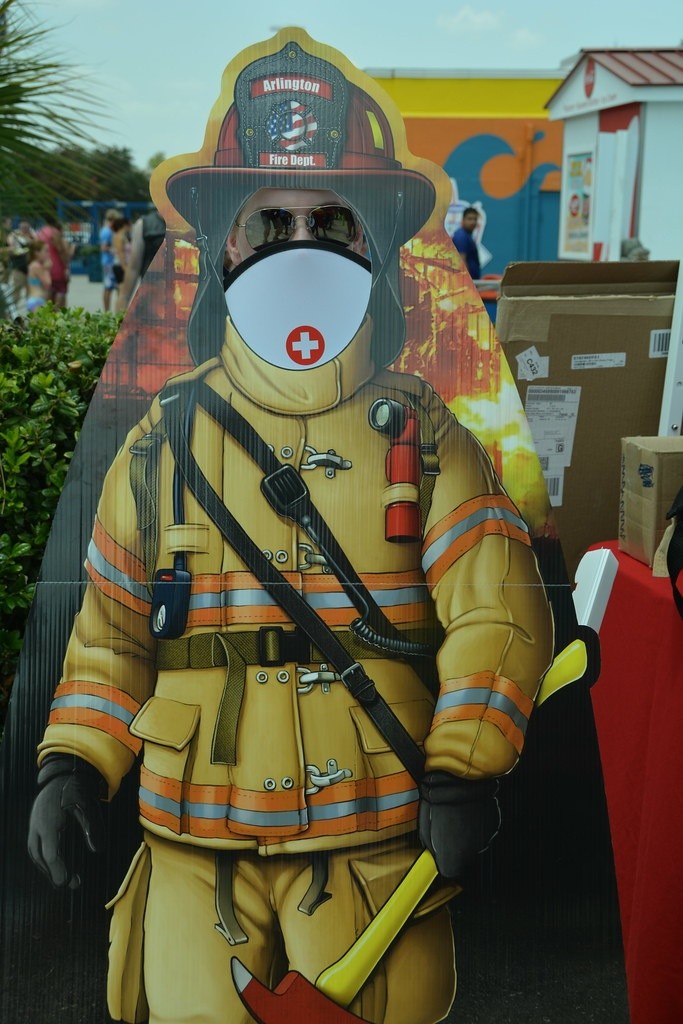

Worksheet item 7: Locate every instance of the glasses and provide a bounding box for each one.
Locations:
[236,205,360,252]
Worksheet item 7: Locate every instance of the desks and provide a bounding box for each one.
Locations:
[584,541,683,1024]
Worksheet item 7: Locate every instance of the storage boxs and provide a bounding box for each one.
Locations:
[618,435,683,569]
[493,261,680,591]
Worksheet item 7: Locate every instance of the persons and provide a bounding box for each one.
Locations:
[0,207,166,314]
[228,187,364,270]
[449,207,484,280]
